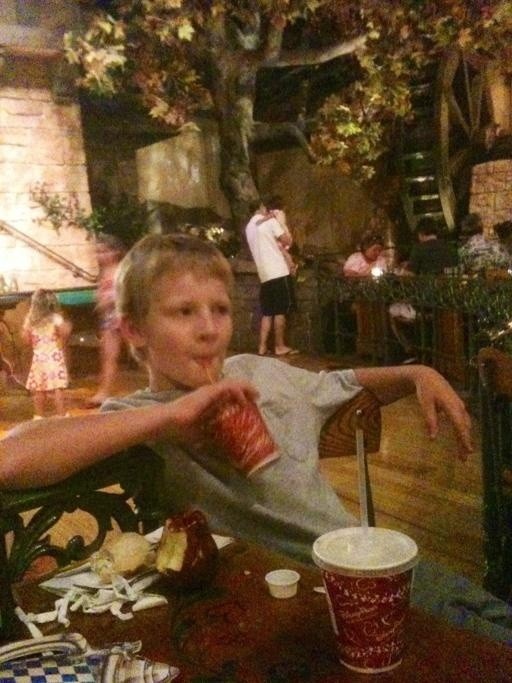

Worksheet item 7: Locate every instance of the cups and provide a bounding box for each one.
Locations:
[312,522,420,675]
[200,393,283,482]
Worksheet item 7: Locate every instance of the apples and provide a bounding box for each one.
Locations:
[156,510,218,590]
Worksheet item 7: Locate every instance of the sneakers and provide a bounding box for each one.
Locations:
[399,353,418,364]
[290,263,299,278]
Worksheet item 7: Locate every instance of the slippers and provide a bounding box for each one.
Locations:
[256,345,301,358]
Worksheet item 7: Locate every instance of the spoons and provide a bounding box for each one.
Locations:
[74,567,157,591]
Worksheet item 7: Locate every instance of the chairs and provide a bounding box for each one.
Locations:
[314,389,381,526]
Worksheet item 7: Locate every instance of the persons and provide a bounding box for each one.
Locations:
[255,192,300,277]
[2,232,512,643]
[446,212,512,272]
[80,231,155,410]
[244,197,295,356]
[339,233,393,357]
[386,214,468,364]
[21,285,76,421]
